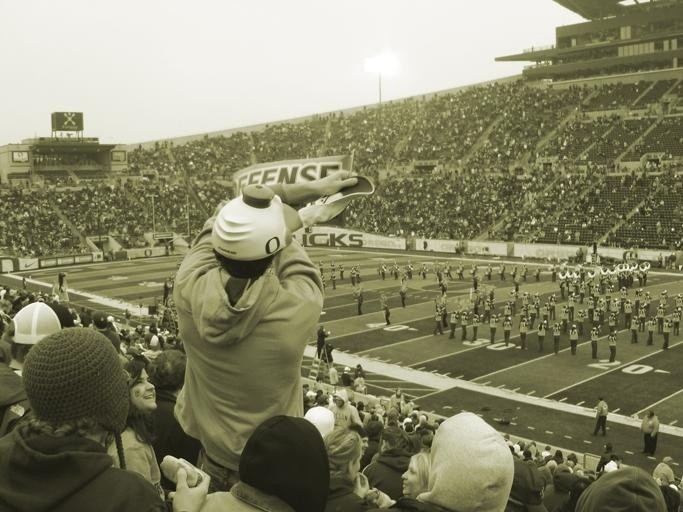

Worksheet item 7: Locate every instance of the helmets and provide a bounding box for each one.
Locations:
[212,186,303,261]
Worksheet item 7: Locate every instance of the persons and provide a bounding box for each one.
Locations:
[0,2,683,512]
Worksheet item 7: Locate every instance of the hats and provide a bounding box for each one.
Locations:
[23,328,129,431]
[10,302,62,346]
